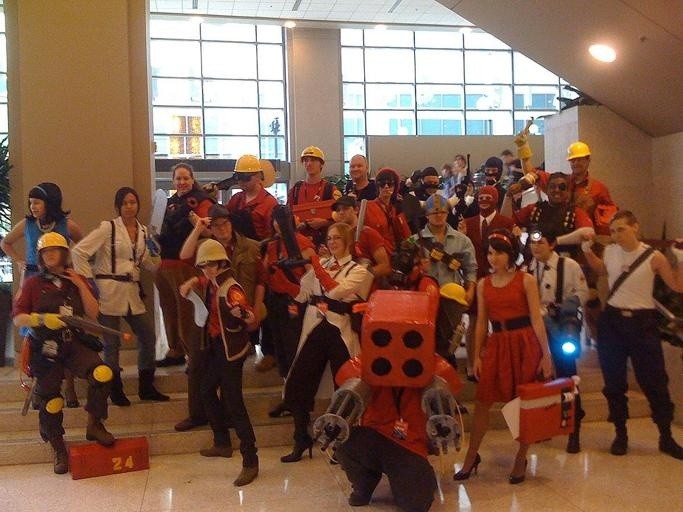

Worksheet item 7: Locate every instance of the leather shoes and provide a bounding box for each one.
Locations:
[65,391,78,408]
[509,458,527,484]
[256,356,277,371]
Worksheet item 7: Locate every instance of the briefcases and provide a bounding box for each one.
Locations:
[514,374,576,444]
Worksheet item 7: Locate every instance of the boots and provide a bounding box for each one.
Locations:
[611,423,627,455]
[110,368,131,405]
[657,422,682,460]
[86,410,115,445]
[138,368,170,401]
[32,435,68,474]
[567,419,580,452]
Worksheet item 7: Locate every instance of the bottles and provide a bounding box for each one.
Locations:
[149,225,159,256]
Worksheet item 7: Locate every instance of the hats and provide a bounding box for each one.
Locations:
[330,195,355,211]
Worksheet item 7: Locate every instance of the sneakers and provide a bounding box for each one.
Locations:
[199,445,233,457]
[349,472,383,506]
[175,417,205,431]
[156,356,187,367]
[268,402,291,418]
[233,466,259,487]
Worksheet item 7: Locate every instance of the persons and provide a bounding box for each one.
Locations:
[12,232,114,474]
[155,130,683,510]
[72,187,170,407]
[1,182,84,410]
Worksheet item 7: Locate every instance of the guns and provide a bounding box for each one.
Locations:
[458,154,472,194]
[58,316,132,342]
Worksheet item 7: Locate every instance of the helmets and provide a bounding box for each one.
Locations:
[440,282,469,307]
[566,142,591,161]
[424,193,449,216]
[36,232,69,256]
[234,154,263,173]
[195,238,233,269]
[300,146,326,166]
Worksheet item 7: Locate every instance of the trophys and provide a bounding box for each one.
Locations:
[514,120,533,145]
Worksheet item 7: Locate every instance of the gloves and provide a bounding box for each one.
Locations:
[258,265,300,298]
[311,255,337,292]
[30,312,69,331]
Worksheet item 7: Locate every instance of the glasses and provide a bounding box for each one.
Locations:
[377,179,395,189]
[236,173,255,182]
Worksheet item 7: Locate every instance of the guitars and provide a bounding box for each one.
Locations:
[205,160,276,194]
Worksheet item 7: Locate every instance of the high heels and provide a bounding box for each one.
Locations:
[452,453,481,481]
[281,435,314,463]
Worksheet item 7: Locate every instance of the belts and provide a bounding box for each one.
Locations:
[606,306,654,318]
